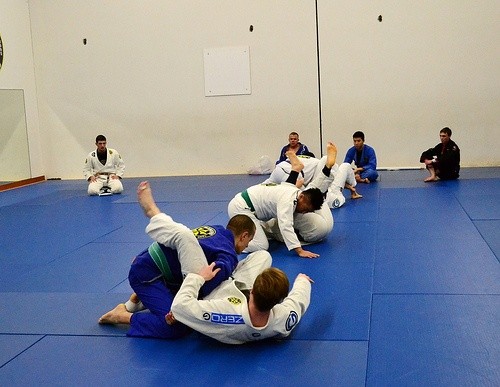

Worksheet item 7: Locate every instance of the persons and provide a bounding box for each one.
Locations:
[227,141,363,258]
[276,132,310,165]
[420,127,460,182]
[99,180,314,344]
[344,131,379,183]
[83,135,125,196]
